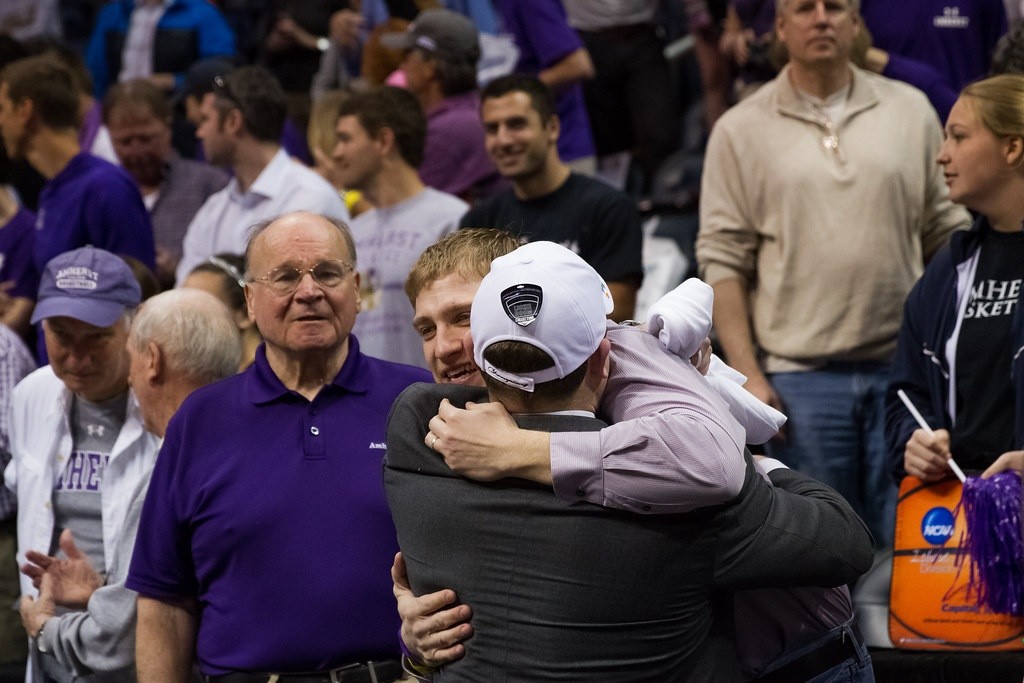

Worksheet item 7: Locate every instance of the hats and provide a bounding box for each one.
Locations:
[31,245,142,328]
[379,8,480,64]
[469,240,614,393]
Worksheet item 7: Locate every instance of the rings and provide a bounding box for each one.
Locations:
[431,436,440,450]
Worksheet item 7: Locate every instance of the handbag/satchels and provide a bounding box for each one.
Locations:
[309,27,371,170]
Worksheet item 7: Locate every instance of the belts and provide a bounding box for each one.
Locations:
[202,659,404,683]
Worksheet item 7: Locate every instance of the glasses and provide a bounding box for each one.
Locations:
[244,259,358,292]
[214,74,244,114]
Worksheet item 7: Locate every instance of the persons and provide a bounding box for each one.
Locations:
[356,230,886,683]
[883,75,1024,681]
[127,207,442,682]
[1,0,784,345]
[0,243,227,683]
[697,0,973,551]
